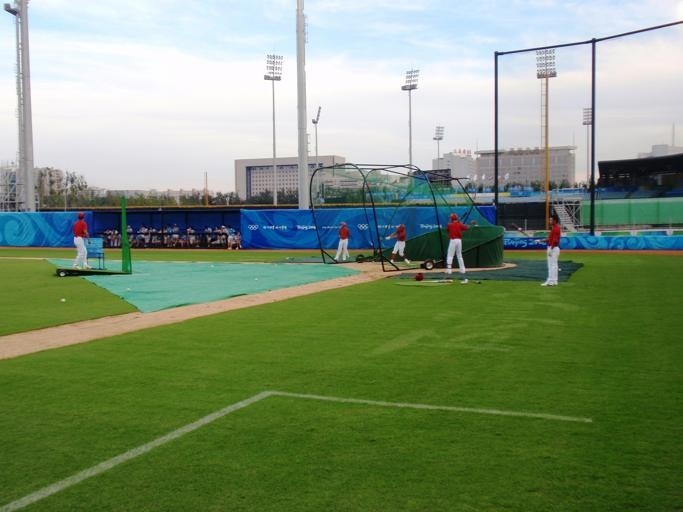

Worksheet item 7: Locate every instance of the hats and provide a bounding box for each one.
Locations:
[400,223,405,229]
[448,213,459,219]
[342,222,347,226]
[78,211,87,219]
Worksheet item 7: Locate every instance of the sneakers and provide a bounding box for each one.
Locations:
[540,280,555,286]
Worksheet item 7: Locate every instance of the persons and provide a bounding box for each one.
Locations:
[385,224,410,264]
[445,214,469,274]
[541,215,560,287]
[73,213,92,270]
[100,225,243,250]
[339,233,349,258]
[334,222,349,261]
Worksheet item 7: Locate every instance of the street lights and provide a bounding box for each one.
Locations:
[262,53,281,207]
[533,47,556,189]
[433,123,444,169]
[581,105,593,185]
[399,67,419,169]
[311,104,321,195]
